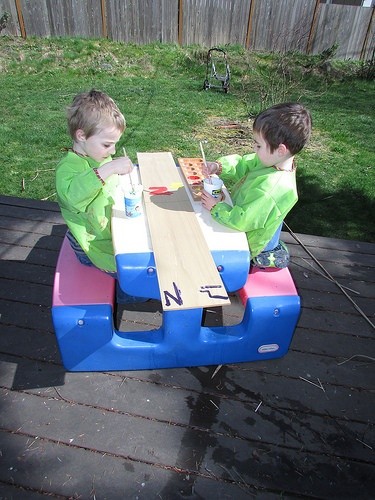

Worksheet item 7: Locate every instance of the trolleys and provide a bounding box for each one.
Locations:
[204,47,231,93]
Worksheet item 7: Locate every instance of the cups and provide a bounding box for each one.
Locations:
[203,177,223,198]
[124,191,141,217]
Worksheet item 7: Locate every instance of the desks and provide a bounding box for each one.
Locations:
[110,166,250,341]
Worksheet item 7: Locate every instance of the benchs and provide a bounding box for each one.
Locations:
[238,239,301,363]
[52,228,115,372]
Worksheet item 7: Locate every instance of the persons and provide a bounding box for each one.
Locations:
[199,101,312,268]
[55,89,152,304]
